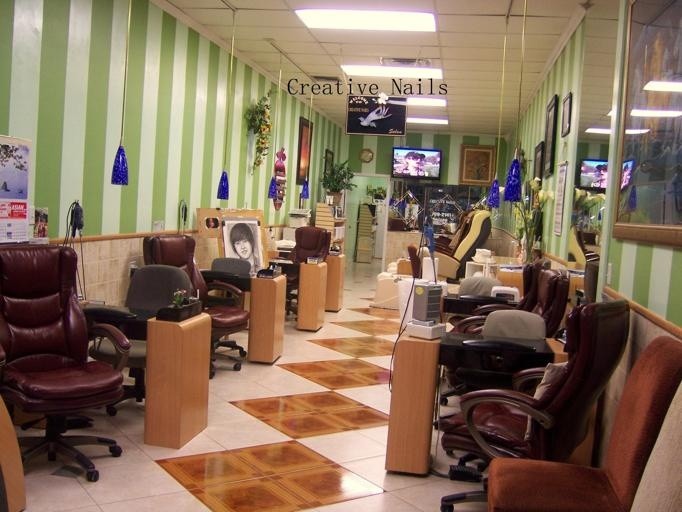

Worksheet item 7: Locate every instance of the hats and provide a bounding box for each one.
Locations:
[405,152,425,158]
[596,164,607,169]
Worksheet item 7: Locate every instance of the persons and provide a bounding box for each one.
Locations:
[393,151,429,176]
[591,164,608,189]
[229,222,261,276]
[359,104,392,127]
[620,164,633,188]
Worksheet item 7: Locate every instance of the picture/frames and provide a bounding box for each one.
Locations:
[295,115,314,186]
[531,91,574,188]
[344,94,407,137]
[324,148,334,184]
[459,143,496,186]
[610,0,682,246]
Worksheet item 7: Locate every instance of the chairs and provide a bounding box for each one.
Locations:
[487,337,682,511]
[439,270,571,406]
[257,227,331,321]
[88,265,193,416]
[433,299,631,510]
[143,234,247,379]
[442,263,550,333]
[407,209,492,285]
[211,257,251,275]
[2,242,131,481]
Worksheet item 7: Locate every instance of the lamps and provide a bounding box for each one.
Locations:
[216,9,238,200]
[299,80,312,199]
[486,1,528,210]
[109,0,133,187]
[268,51,284,200]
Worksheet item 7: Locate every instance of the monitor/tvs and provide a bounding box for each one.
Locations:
[392,146,443,180]
[579,158,609,190]
[621,159,635,189]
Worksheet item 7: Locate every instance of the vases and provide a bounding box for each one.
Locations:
[575,229,587,252]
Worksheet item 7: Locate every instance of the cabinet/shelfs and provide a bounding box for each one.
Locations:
[356,203,377,263]
[314,201,347,256]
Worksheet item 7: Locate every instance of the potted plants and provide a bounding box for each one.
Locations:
[322,156,358,205]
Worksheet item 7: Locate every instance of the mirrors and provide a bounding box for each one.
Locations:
[565,0,621,271]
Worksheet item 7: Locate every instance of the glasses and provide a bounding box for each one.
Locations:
[407,157,424,161]
[598,169,607,173]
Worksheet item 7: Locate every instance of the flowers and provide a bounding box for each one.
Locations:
[513,178,550,264]
[243,91,273,174]
[574,187,606,235]
